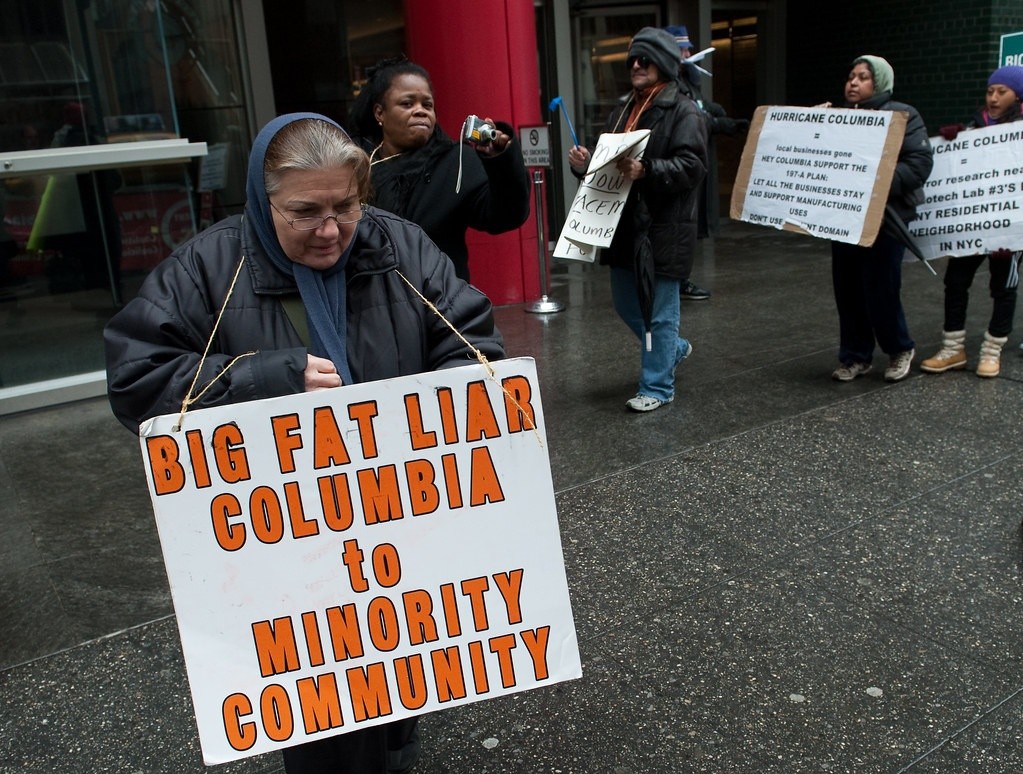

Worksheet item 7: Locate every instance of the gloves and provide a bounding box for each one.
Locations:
[940,126,958,141]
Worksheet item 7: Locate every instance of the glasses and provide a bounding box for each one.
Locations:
[627,55,655,69]
[269,197,366,230]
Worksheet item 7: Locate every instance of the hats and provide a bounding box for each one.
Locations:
[665,25,695,47]
[987,66,1023,101]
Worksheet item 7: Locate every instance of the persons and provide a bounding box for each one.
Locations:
[833,54,933,380]
[568,26,711,411]
[365,55,533,283]
[664,27,734,299]
[920,66,1023,376]
[102,111,506,774]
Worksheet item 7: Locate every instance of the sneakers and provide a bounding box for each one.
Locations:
[832,360,872,381]
[625,392,674,411]
[885,347,915,381]
[672,344,693,374]
[678,281,711,300]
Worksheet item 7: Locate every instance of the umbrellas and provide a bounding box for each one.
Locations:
[884,205,938,277]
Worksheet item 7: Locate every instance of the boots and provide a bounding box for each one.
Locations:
[921,330,968,371]
[976,331,1008,377]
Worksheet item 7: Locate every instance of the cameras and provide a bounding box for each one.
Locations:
[465,115,497,147]
